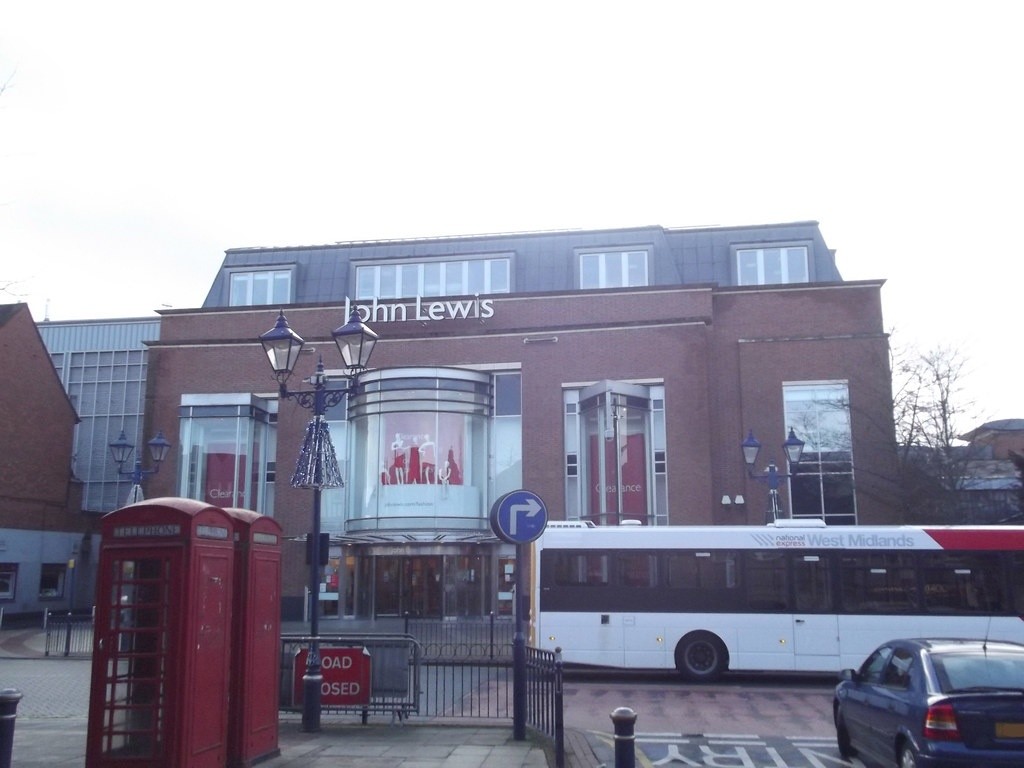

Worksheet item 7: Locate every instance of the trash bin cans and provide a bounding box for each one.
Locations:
[0,688,24,768]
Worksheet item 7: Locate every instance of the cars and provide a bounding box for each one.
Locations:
[832,636,1024,768]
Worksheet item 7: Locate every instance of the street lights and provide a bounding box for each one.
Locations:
[257,300,380,736]
[738,426,805,521]
[107,425,173,505]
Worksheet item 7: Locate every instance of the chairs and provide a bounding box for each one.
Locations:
[967,657,1011,688]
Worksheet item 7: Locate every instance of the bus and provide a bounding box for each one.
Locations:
[527,519,1024,686]
